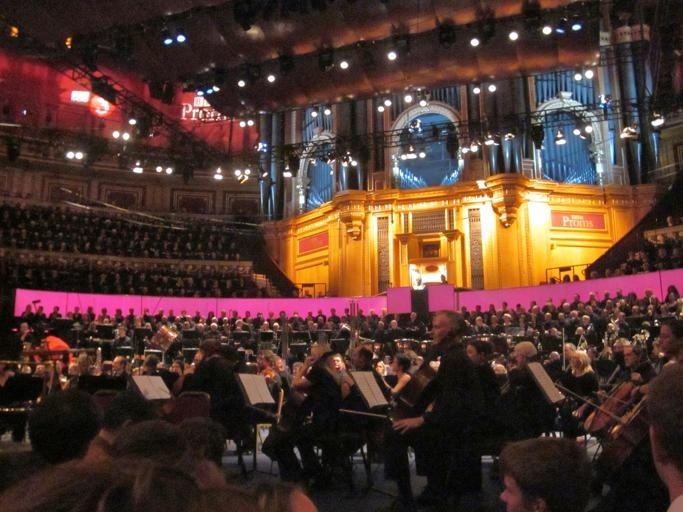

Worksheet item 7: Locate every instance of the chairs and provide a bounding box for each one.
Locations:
[92,381,543,511]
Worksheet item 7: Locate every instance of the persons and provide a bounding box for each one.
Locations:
[0,305,435,488]
[1,388,317,512]
[393,309,480,510]
[649,364,683,512]
[463,288,683,512]
[0,196,294,297]
[543,212,682,287]
[500,437,591,512]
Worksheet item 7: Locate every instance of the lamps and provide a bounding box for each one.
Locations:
[0,1,683,181]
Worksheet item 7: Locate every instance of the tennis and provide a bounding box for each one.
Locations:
[581,362,650,453]
[394,359,440,415]
